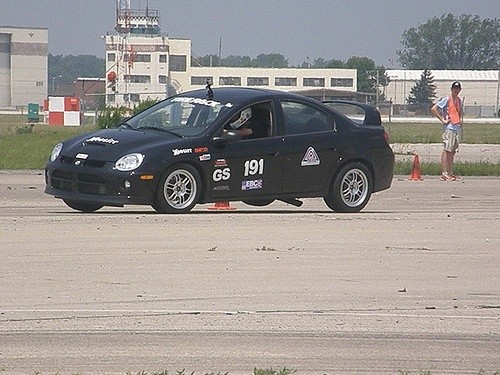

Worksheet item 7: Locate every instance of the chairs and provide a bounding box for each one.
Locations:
[253,106,271,139]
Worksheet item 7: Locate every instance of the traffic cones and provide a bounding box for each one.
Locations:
[206,201,235,211]
[408,154,424,181]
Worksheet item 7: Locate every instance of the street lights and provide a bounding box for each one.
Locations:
[52,75,62,93]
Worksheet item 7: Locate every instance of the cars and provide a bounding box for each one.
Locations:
[43,86,395,214]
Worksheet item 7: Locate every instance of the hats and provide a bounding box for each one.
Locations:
[452,82,461,88]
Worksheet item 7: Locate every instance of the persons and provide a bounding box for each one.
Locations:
[432,81,464,181]
[225,109,262,138]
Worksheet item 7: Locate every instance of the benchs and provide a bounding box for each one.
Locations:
[283,111,330,134]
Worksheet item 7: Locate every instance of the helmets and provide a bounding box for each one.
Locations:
[228,107,252,130]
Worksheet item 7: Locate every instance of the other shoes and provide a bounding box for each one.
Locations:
[450,175,462,180]
[439,174,455,181]
